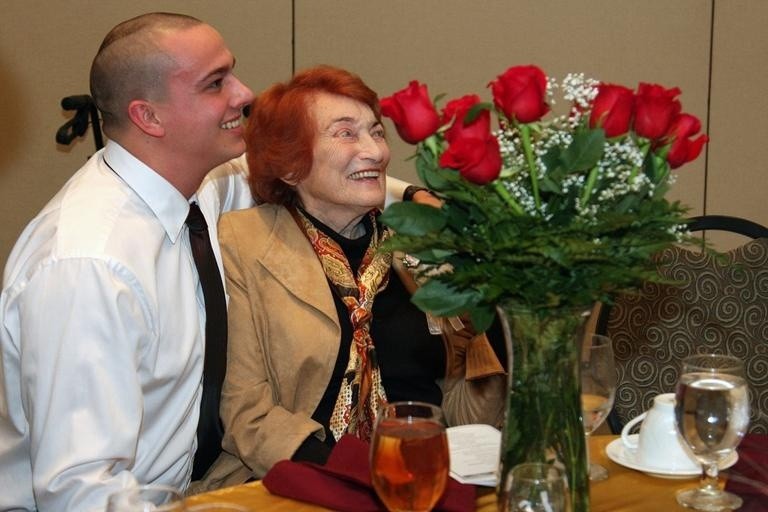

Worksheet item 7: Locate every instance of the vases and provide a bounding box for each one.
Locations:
[492,295,595,509]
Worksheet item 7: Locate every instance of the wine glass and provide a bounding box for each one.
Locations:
[676,353,752,511]
[579,330,614,482]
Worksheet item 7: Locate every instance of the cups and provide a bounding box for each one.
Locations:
[619,391,702,471]
[369,400,451,511]
[503,463,572,510]
[102,486,190,511]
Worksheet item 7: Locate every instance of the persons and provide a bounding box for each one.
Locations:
[189,64,508,495]
[0,12,445,512]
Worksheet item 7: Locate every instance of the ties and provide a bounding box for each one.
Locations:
[184,200,226,482]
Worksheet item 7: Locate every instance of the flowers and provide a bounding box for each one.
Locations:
[371,63,710,507]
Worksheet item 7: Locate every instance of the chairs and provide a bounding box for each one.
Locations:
[604,216,767,499]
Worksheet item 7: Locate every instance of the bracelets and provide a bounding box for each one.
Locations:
[403,186,441,202]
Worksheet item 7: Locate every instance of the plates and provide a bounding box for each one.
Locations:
[606,434,742,479]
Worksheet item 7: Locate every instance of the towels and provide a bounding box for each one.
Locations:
[264,431,479,511]
